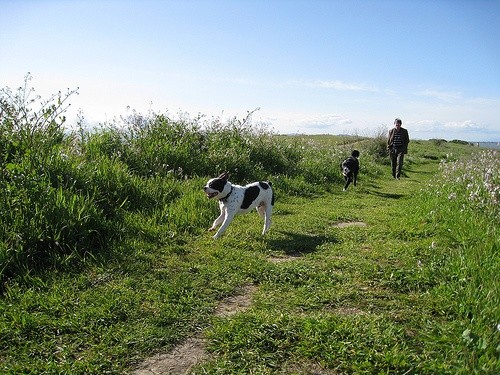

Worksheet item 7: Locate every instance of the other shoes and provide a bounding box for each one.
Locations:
[354,184,357,189]
[343,186,346,192]
[396,172,400,181]
[391,174,396,179]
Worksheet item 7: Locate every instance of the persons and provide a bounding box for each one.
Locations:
[387,119,409,181]
[340,149,360,191]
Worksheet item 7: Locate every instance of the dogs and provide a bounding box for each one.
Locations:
[203,171,275,241]
